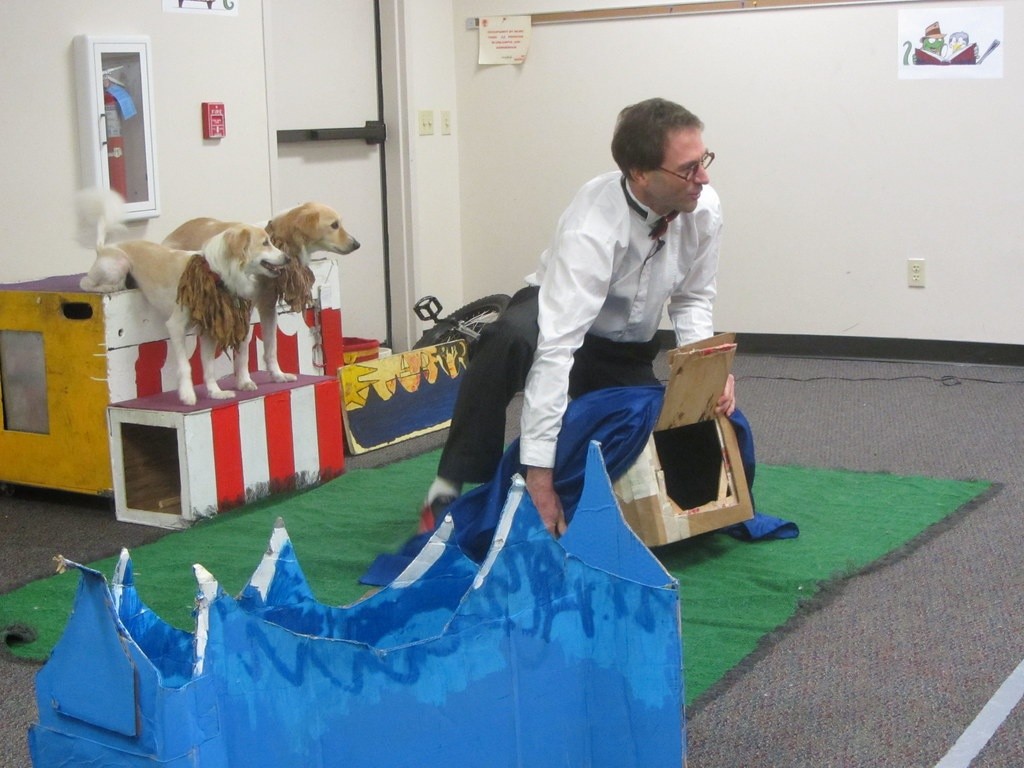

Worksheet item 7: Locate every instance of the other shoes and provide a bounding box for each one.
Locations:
[417,487,459,534]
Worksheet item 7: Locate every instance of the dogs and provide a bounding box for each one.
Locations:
[70,185,293,407]
[160,199,361,393]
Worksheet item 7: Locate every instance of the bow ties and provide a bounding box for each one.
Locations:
[648,210,680,240]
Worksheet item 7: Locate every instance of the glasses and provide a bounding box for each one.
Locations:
[656,152,716,181]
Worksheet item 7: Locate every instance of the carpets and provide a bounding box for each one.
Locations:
[0,445,1003,725]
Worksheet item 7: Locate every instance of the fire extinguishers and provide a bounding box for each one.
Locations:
[102,65,129,203]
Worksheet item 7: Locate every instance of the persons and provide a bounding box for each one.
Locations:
[419,98,736,539]
